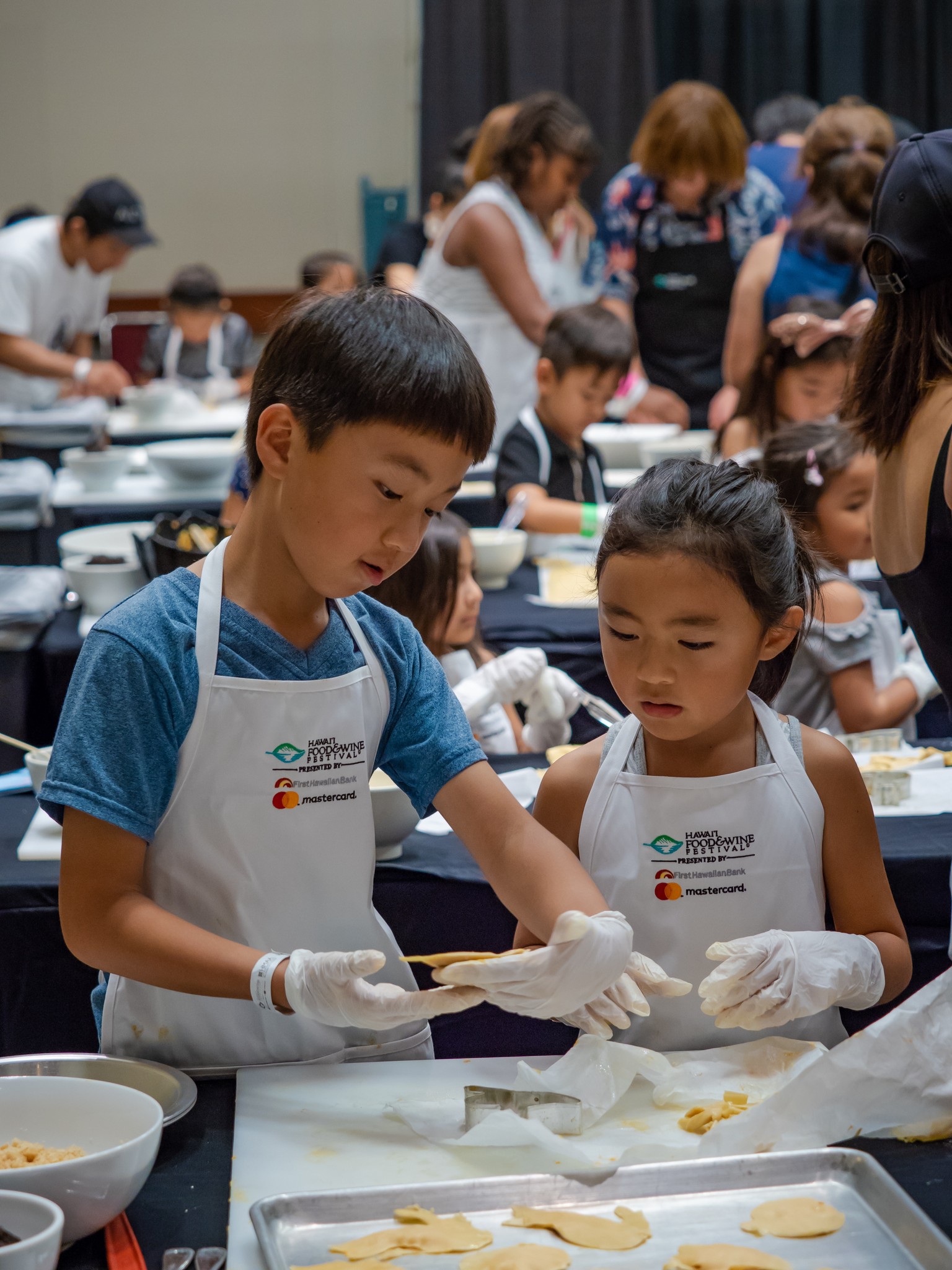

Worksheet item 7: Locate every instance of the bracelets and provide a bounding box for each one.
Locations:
[604,369,649,420]
[72,356,94,388]
[580,501,597,537]
[248,947,295,1017]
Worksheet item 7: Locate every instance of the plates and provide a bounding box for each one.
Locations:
[0,1053,202,1130]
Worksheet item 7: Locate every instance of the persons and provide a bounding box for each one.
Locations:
[702,297,875,464]
[586,82,795,432]
[719,97,897,419]
[446,124,478,201]
[298,254,358,299]
[488,303,628,536]
[41,281,636,1080]
[464,103,596,285]
[507,456,916,1048]
[132,263,259,403]
[851,126,952,748]
[360,507,578,755]
[401,90,691,454]
[739,421,943,742]
[748,94,823,220]
[0,178,164,409]
[365,161,472,295]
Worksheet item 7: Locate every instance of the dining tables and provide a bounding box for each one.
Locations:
[0,1040,952,1270]
[0,739,951,944]
[0,372,943,726]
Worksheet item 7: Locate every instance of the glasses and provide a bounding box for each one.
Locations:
[555,141,606,168]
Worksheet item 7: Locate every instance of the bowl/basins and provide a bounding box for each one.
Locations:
[466,523,528,591]
[0,1185,66,1270]
[0,1073,166,1255]
[60,546,156,615]
[118,380,171,420]
[145,436,240,492]
[56,520,161,563]
[362,760,427,861]
[61,445,131,492]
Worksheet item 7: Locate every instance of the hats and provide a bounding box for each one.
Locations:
[73,180,159,253]
[858,124,952,302]
[165,264,222,299]
